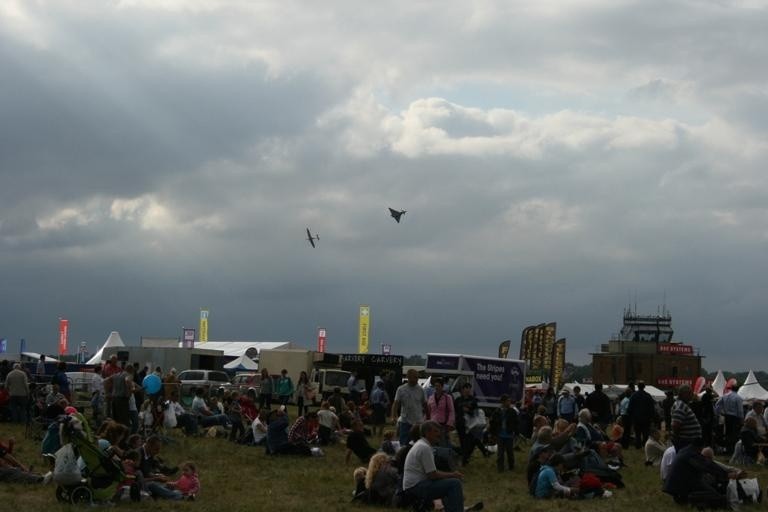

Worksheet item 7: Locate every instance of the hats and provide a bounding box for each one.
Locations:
[532,443,551,455]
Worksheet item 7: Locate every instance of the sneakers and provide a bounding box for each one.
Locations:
[42,471,53,487]
[185,494,196,501]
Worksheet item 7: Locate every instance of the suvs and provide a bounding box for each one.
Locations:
[230,371,281,400]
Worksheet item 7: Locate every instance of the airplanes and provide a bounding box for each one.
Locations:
[305,228,320,250]
[387,207,408,223]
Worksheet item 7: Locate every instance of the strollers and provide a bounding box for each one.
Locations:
[41,403,144,506]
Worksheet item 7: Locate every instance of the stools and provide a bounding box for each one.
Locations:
[408,495,433,512]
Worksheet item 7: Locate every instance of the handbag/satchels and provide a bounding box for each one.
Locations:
[306,387,316,400]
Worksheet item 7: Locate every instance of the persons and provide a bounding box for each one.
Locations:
[1,353,768,512]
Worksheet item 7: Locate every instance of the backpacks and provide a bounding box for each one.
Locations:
[671,444,698,473]
[375,472,398,507]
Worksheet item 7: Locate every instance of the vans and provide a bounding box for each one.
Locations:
[175,370,231,391]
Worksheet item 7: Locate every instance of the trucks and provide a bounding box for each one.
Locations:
[257,347,354,405]
[422,352,528,415]
